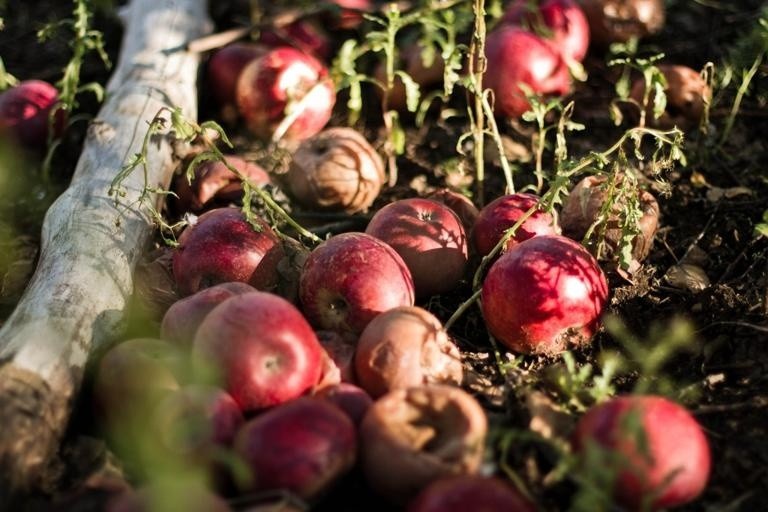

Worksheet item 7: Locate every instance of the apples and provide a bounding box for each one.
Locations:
[191,292,320,412]
[173,208,285,304]
[357,307,462,388]
[578,395,710,511]
[162,282,260,351]
[379,37,450,119]
[326,0,371,36]
[301,234,415,342]
[367,200,467,292]
[173,155,267,222]
[238,48,336,144]
[631,64,707,131]
[423,479,528,512]
[209,52,270,120]
[283,18,329,51]
[469,27,571,124]
[292,131,377,217]
[99,339,190,427]
[603,0,662,41]
[473,198,558,274]
[1,83,55,140]
[232,397,353,498]
[483,234,608,363]
[362,385,486,496]
[156,386,239,461]
[563,174,658,264]
[507,0,587,61]
